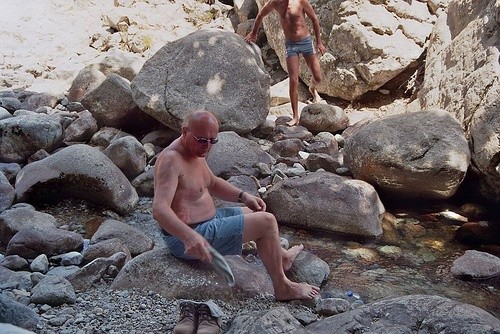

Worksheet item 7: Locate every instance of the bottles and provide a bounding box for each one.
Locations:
[343,290,363,310]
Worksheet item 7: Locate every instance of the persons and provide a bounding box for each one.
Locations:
[152,111,320,299]
[244,0,325,126]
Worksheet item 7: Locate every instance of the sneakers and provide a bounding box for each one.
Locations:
[173,302,197,334]
[197,304,222,334]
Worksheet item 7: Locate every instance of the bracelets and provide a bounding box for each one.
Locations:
[238,192,243,202]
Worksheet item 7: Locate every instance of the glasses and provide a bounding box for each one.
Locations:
[187,128,219,144]
